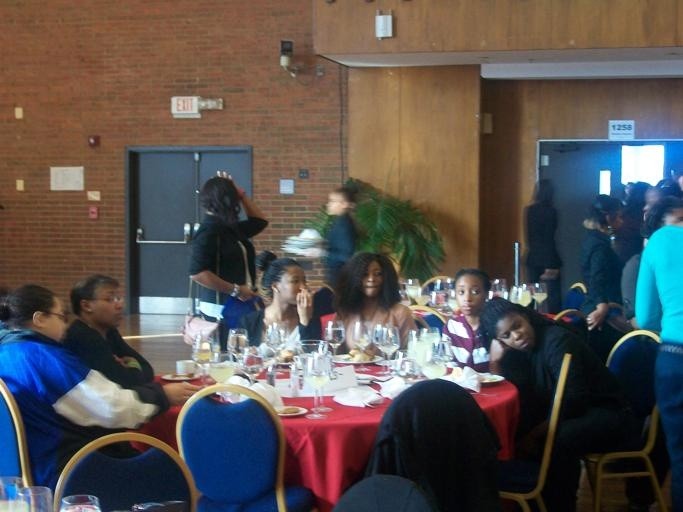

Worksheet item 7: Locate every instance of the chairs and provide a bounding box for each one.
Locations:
[175,383,316,512]
[421,276,458,294]
[565,282,588,307]
[500,353,571,512]
[51,432,197,512]
[0,377,39,512]
[220,297,265,331]
[551,309,586,324]
[365,379,500,512]
[307,282,338,321]
[581,329,669,512]
[410,303,447,337]
[607,302,623,321]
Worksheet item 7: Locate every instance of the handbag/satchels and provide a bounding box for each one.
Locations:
[182,313,218,348]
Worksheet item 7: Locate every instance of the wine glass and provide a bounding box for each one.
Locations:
[305,353,334,420]
[374,324,400,375]
[509,281,548,308]
[227,328,250,371]
[406,327,455,368]
[266,322,286,357]
[324,320,346,358]
[352,320,372,358]
[406,279,421,306]
[490,278,507,299]
[192,330,216,389]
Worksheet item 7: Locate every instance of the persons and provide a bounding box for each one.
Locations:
[297,186,358,290]
[440,268,491,374]
[480,170,683,511]
[57,273,154,459]
[188,169,269,352]
[239,251,322,353]
[0,284,205,512]
[320,251,418,350]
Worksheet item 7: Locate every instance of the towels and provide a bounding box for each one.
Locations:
[455,365,481,393]
[334,385,384,408]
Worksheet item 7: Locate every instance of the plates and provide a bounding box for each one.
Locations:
[161,375,199,381]
[480,373,505,384]
[277,406,307,417]
[333,354,383,364]
[280,236,323,253]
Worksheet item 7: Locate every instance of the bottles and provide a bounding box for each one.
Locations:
[431,278,456,305]
[289,364,300,394]
[266,366,275,388]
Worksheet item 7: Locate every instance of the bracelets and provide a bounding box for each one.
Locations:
[127,359,143,370]
[231,283,241,298]
[239,187,247,198]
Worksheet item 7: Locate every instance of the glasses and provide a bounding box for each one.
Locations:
[78,296,125,304]
[39,309,70,322]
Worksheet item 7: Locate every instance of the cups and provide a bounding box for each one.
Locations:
[0,475,101,512]
[176,360,195,376]
[296,340,328,357]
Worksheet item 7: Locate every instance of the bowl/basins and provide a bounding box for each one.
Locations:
[299,229,320,239]
